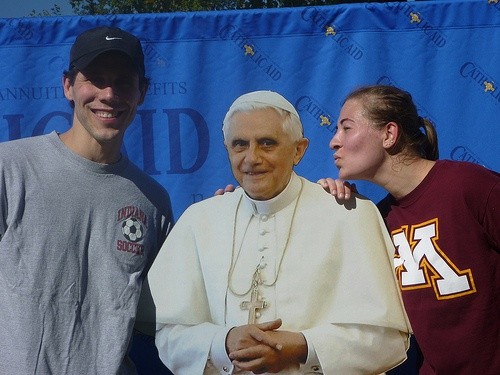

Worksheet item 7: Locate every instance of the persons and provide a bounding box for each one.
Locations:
[0,26,234,375]
[146,90,413,375]
[316,77,499,375]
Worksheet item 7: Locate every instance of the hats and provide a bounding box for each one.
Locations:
[69,27,146,80]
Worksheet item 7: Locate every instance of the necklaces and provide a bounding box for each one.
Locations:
[227,178,304,325]
